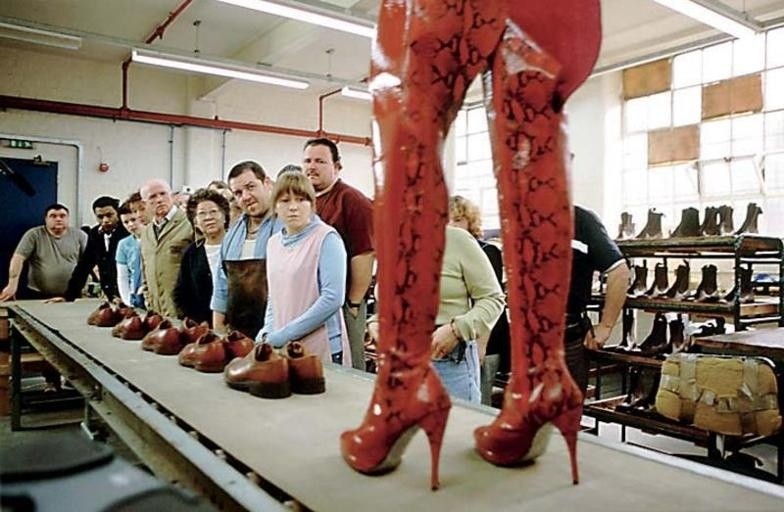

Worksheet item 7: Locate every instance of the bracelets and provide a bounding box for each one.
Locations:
[450,319,460,340]
[345,299,360,309]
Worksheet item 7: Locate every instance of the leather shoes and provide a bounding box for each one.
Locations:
[88,302,327,398]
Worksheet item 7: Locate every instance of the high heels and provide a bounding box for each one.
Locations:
[342,366,505,490]
[475,368,601,483]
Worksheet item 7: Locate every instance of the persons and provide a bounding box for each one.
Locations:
[254,170,353,367]
[338,0,602,493]
[563,205,630,401]
[430,196,511,403]
[3,203,99,301]
[44,165,303,329]
[210,161,322,341]
[301,138,376,372]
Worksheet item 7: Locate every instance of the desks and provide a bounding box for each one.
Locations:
[0,295,784,511]
[696,323,783,481]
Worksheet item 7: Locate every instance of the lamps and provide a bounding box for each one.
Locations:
[651,1,766,39]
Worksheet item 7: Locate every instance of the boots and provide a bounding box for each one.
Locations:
[616,367,661,416]
[615,202,763,241]
[618,262,755,359]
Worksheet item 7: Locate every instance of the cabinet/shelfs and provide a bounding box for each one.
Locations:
[584,232,783,447]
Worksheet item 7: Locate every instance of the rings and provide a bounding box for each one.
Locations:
[440,349,447,356]
